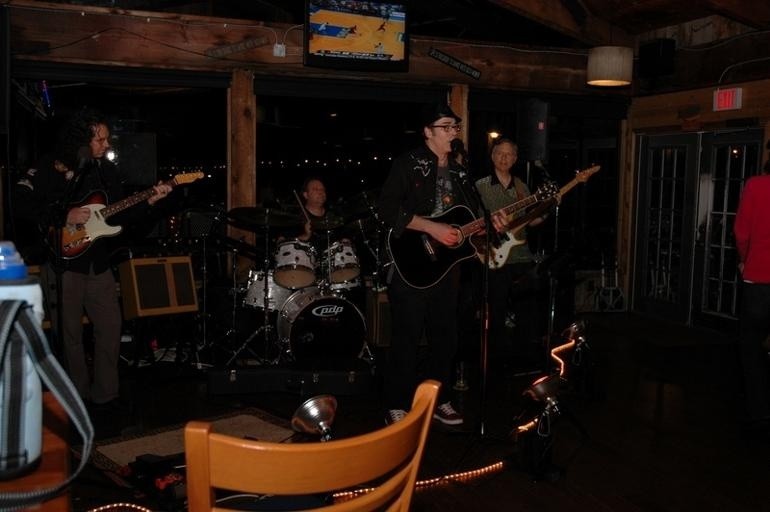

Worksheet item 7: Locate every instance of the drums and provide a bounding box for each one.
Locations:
[321,238,362,283]
[243,268,292,311]
[276,285,368,374]
[273,239,319,290]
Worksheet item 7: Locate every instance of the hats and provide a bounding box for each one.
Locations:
[421,104,462,129]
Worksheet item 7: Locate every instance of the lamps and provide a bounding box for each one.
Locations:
[529,376,560,414]
[586,43,634,87]
[567,317,589,351]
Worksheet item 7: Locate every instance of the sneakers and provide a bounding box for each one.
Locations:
[385,410,408,426]
[433,401,463,425]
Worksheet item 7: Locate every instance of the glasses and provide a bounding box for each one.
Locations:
[429,125,462,131]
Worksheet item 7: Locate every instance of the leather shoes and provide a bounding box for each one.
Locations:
[94,397,135,411]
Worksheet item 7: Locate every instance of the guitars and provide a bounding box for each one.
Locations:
[35,166,205,260]
[382,182,563,292]
[475,161,604,272]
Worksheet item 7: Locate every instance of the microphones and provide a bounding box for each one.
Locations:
[451,138,473,164]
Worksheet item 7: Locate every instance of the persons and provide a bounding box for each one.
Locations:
[374,22,388,34]
[734,142,770,435]
[476,137,563,379]
[379,106,510,424]
[15,113,172,440]
[273,178,348,244]
[375,43,384,57]
[344,25,360,39]
[318,22,328,32]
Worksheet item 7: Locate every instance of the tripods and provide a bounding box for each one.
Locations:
[179,246,221,366]
[518,207,585,405]
[208,246,257,365]
[229,213,299,370]
[439,209,535,484]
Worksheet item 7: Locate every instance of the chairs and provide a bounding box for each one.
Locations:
[183,380,442,510]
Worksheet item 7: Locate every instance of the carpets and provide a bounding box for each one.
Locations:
[69,406,303,507]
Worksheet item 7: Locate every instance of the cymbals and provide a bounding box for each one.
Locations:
[188,228,270,264]
[228,203,303,231]
